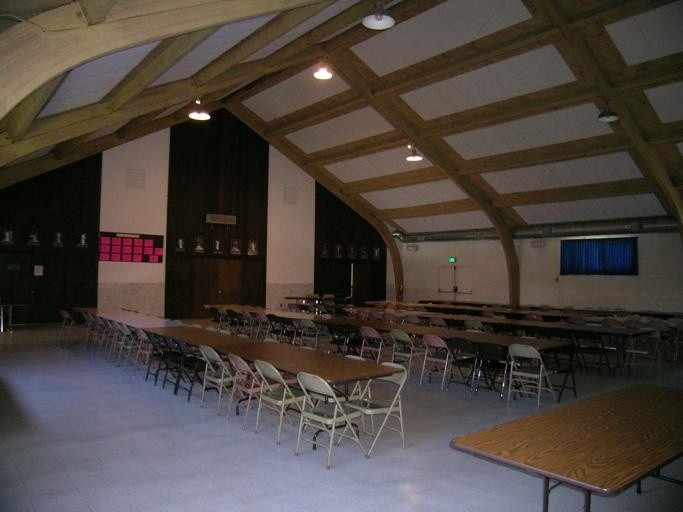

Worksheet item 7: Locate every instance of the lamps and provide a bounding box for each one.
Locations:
[595,105,620,124]
[187,104,212,123]
[360,2,397,32]
[404,149,424,163]
[311,64,334,81]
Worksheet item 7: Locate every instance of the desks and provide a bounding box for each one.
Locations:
[448,382,683,511]
